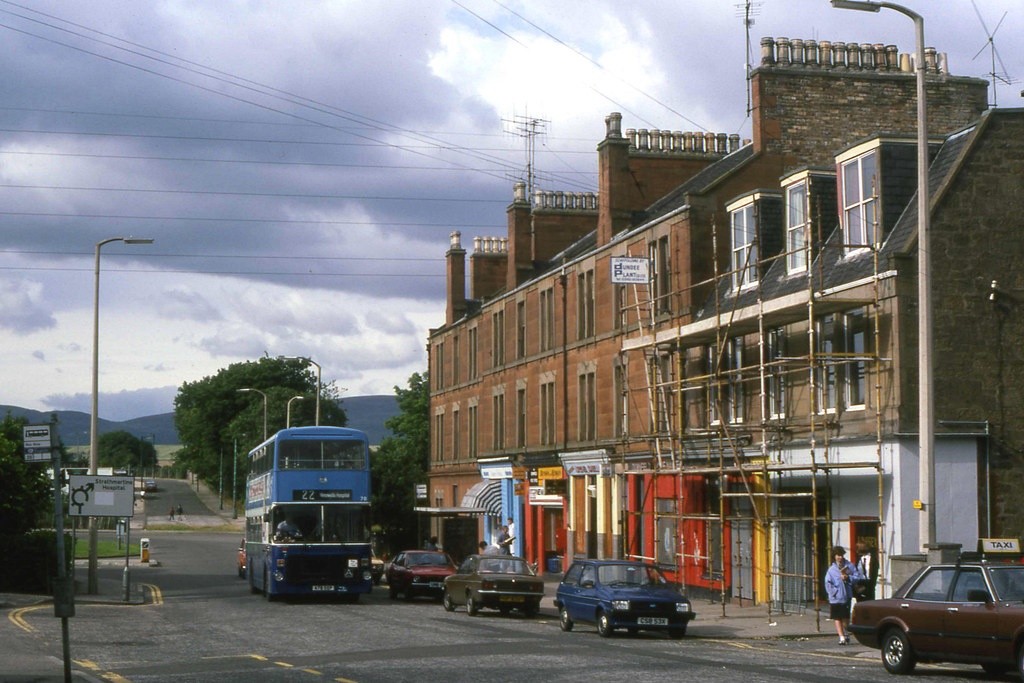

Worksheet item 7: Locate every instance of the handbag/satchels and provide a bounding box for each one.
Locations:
[854,581,870,598]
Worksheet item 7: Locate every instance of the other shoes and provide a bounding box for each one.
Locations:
[839,638,845,644]
[845,634,850,640]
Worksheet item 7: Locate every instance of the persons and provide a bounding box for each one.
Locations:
[428,537,442,551]
[496,525,512,555]
[176,505,182,521]
[479,541,500,571]
[170,507,174,520]
[824,546,860,645]
[857,548,879,601]
[507,518,514,555]
[276,513,299,539]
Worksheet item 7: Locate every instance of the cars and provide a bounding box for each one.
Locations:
[553,559,697,640]
[238,538,246,578]
[845,538,1024,681]
[442,554,546,619]
[370,549,384,584]
[385,550,458,601]
[146,482,156,491]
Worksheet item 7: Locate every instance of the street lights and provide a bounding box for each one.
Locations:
[283,355,321,426]
[238,388,266,442]
[285,396,304,469]
[831,0,938,553]
[88,237,154,595]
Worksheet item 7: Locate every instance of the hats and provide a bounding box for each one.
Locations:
[832,547,847,555]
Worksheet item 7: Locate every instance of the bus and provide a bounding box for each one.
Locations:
[245,426,372,600]
